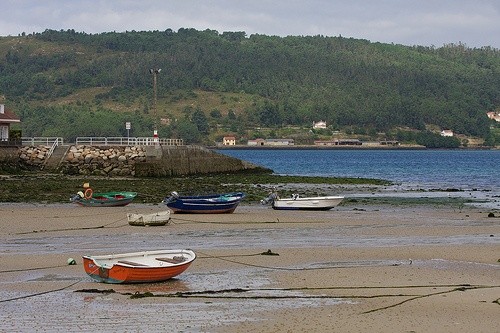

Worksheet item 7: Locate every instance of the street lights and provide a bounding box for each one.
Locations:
[148,68,163,145]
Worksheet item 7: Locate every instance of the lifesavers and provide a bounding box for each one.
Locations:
[85,189,93,199]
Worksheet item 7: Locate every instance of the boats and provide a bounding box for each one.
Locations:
[261,191,345,211]
[169,190,246,215]
[82,248,196,284]
[70,190,138,208]
[126,211,171,227]
